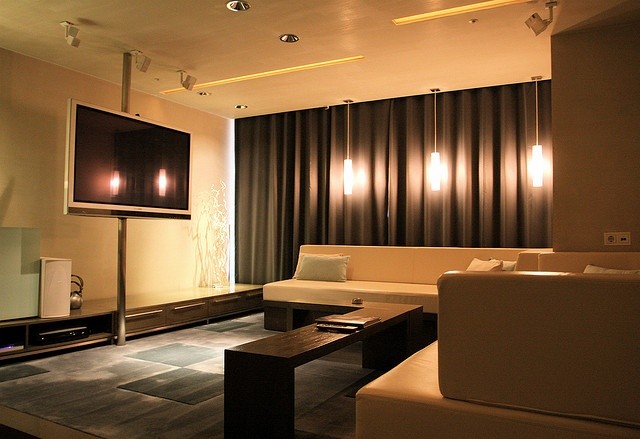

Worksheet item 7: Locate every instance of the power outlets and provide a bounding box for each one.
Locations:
[604,232,631,245]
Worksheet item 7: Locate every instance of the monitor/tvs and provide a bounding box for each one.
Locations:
[63,98,193,220]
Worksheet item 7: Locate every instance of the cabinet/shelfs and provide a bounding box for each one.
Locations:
[0,281,264,362]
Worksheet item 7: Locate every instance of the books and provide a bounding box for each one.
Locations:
[315,313,381,327]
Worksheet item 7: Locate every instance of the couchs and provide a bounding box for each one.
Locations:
[355,252,640,439]
[262,244,552,333]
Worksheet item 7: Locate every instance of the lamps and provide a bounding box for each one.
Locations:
[343,99,354,196]
[524,1,557,36]
[430,89,441,190]
[59,20,81,48]
[176,69,197,91]
[158,150,167,197]
[129,50,151,72]
[112,153,120,196]
[531,76,543,188]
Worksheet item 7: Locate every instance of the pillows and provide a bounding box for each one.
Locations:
[487,257,517,271]
[583,263,640,274]
[293,253,342,279]
[466,258,503,272]
[296,255,351,281]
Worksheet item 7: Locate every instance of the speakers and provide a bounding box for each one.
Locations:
[182,75,197,92]
[39,255,73,320]
[136,54,152,73]
